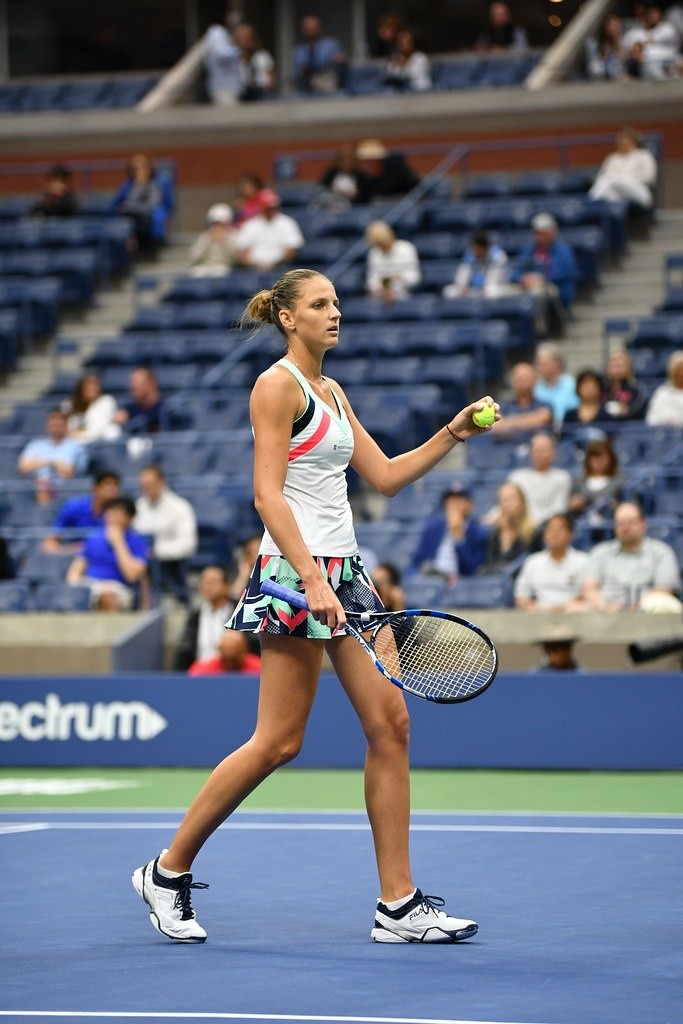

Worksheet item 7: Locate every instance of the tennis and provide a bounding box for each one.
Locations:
[472,402,496,428]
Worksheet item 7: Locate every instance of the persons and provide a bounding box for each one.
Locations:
[0,0,683,677]
[131,267,501,944]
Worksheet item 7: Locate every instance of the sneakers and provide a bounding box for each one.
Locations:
[131,849,211,945]
[369,889,479,944]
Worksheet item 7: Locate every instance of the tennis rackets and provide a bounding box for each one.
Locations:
[257,580,501,703]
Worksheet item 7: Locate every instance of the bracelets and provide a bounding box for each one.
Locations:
[446,423,464,441]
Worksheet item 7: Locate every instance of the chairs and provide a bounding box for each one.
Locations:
[0,49,592,111]
[1,167,683,609]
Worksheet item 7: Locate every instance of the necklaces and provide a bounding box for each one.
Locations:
[287,352,322,384]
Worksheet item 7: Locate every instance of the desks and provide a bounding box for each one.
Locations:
[1,612,168,673]
[414,611,683,675]
